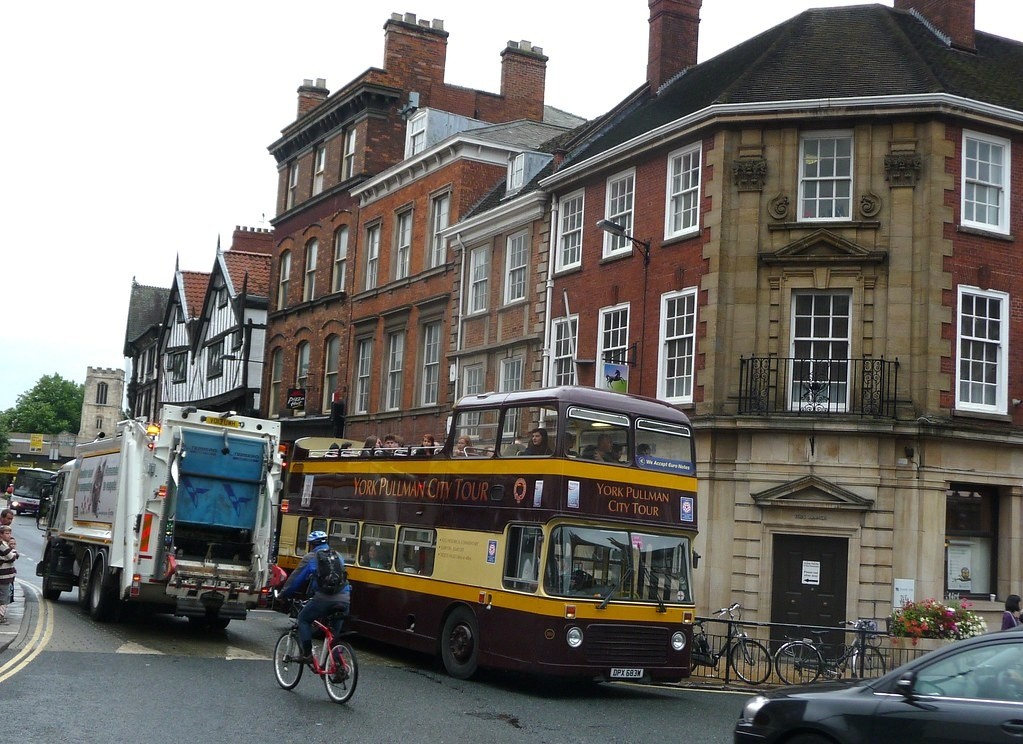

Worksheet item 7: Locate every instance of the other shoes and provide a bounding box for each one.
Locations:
[0,617,9,624]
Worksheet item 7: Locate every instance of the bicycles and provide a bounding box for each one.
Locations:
[691,602,772,685]
[273,589,358,704]
[774,621,886,686]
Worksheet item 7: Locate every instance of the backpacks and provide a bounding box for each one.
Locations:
[306,546,349,595]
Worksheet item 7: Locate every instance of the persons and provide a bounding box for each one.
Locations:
[361,434,400,456]
[278,531,350,683]
[453,435,473,456]
[329,442,352,455]
[20,482,30,492]
[416,434,435,454]
[1001,595,1022,630]
[369,543,383,568]
[636,443,651,456]
[0,509,19,624]
[565,432,622,463]
[520,537,575,590]
[520,428,552,455]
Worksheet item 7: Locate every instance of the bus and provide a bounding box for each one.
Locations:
[9,467,57,515]
[269,384,699,681]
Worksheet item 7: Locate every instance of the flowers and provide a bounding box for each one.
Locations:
[887,598,988,646]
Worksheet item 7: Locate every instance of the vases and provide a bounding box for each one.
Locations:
[885,617,944,640]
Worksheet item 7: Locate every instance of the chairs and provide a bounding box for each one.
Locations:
[453,450,463,457]
[464,446,495,458]
[324,452,339,457]
[503,443,527,457]
[374,451,384,456]
[394,450,408,456]
[311,452,324,457]
[434,449,442,455]
[561,570,597,590]
[341,452,360,458]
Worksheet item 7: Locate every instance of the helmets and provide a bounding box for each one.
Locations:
[306,531,328,542]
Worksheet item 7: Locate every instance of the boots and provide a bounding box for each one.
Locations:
[292,640,314,664]
[331,651,350,683]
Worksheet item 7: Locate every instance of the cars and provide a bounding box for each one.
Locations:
[734,630,1023,744]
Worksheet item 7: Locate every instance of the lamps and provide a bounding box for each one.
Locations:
[595,219,651,267]
[219,355,266,364]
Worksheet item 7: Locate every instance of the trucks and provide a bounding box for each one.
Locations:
[36,404,289,629]
